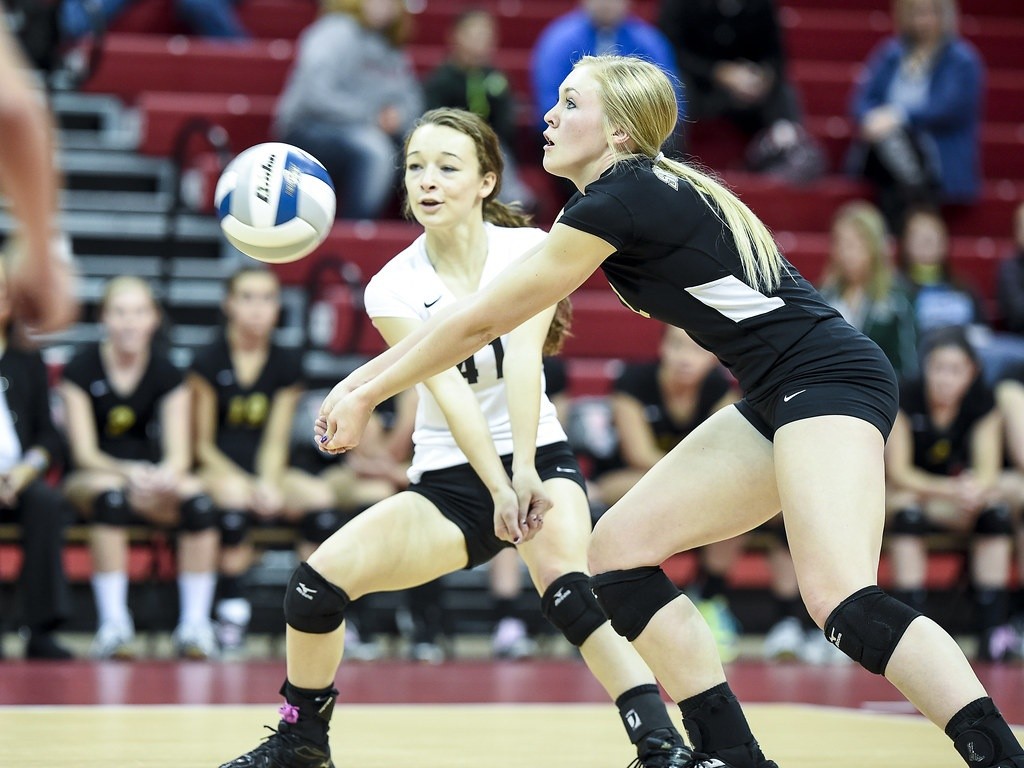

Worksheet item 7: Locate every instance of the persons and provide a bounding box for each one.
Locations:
[214,107,727,768]
[313,55,1024,768]
[0,0,1024,667]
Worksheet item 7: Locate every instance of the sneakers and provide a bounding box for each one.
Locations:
[680,749,779,768]
[219,720,336,768]
[625,729,692,768]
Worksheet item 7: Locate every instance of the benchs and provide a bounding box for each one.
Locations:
[0,0,1024,592]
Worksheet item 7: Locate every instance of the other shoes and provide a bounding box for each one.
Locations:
[96,624,136,661]
[990,629,1021,661]
[24,640,75,661]
[177,622,214,659]
[493,618,527,658]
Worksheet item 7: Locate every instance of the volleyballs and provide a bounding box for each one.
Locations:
[214,142,336,263]
